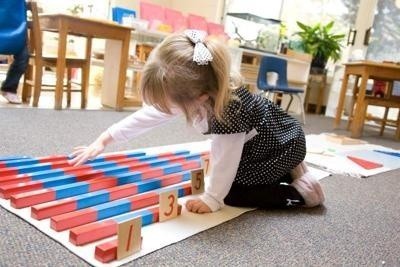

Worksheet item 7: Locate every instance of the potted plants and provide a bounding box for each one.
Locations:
[291,19,346,74]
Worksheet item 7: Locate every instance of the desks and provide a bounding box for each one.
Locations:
[229,45,311,114]
[25,13,135,112]
[305,72,326,114]
[332,61,400,140]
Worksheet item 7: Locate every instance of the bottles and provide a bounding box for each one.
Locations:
[280,39,288,55]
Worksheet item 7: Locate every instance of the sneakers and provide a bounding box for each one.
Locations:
[290,162,309,180]
[1,89,22,104]
[292,170,325,208]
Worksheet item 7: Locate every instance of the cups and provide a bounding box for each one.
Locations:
[266,71,279,86]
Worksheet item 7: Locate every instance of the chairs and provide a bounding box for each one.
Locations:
[256,54,307,126]
[346,61,400,139]
[21,2,89,110]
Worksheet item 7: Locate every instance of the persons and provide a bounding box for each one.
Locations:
[67,28,324,214]
[0,1,32,104]
